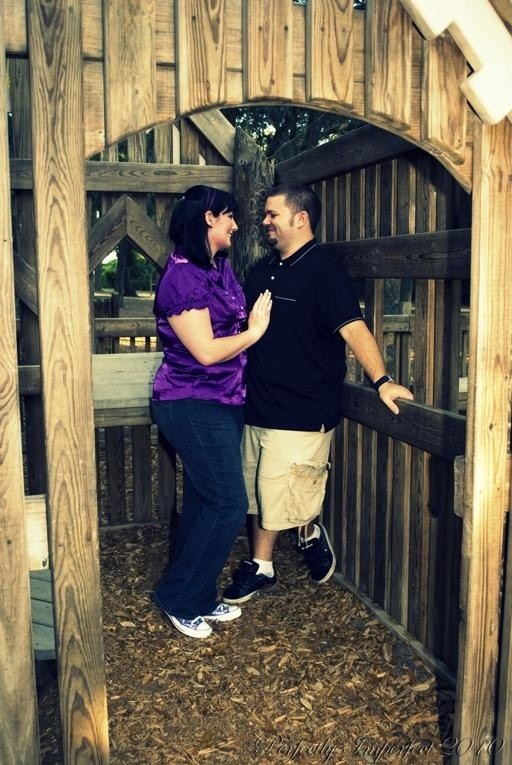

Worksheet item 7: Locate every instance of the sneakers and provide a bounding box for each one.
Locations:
[222,557,278,605]
[201,603,243,622]
[159,606,214,639]
[297,521,337,586]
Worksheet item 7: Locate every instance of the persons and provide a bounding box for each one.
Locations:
[217,180,418,609]
[148,182,275,643]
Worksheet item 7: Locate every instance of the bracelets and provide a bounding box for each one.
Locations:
[371,373,394,396]
